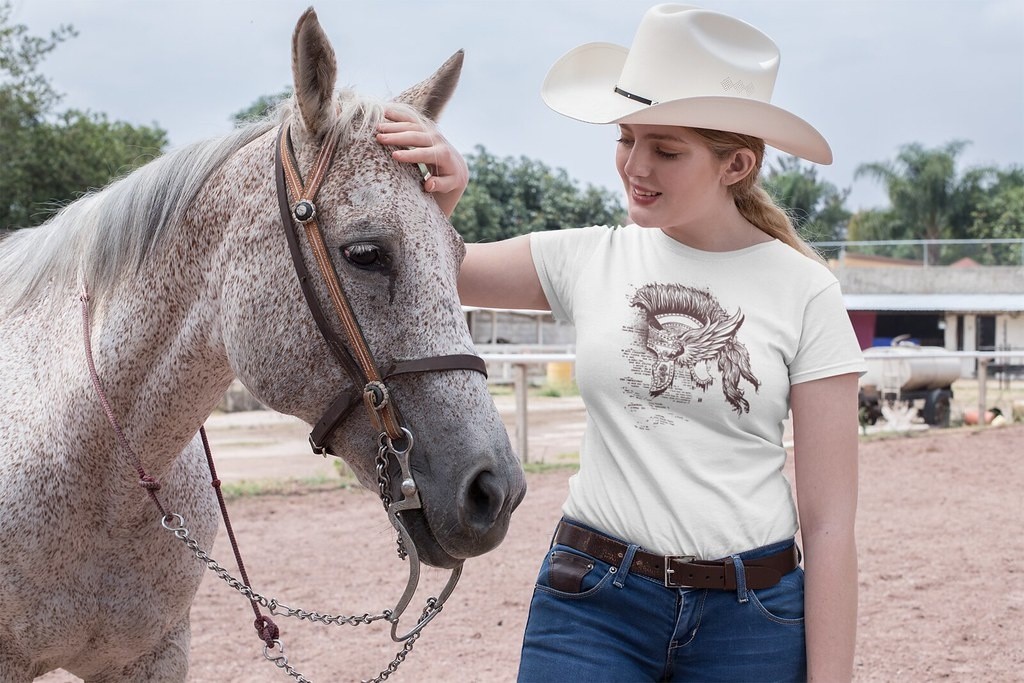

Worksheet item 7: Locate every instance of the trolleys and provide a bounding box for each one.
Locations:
[857,340,961,431]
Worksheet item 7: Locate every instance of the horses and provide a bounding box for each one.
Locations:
[0,6,527,683]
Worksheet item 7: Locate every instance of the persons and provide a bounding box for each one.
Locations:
[375,3,866,683]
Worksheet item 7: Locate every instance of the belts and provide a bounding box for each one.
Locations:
[554,518,803,593]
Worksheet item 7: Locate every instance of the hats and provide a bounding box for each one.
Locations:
[541,2,837,171]
